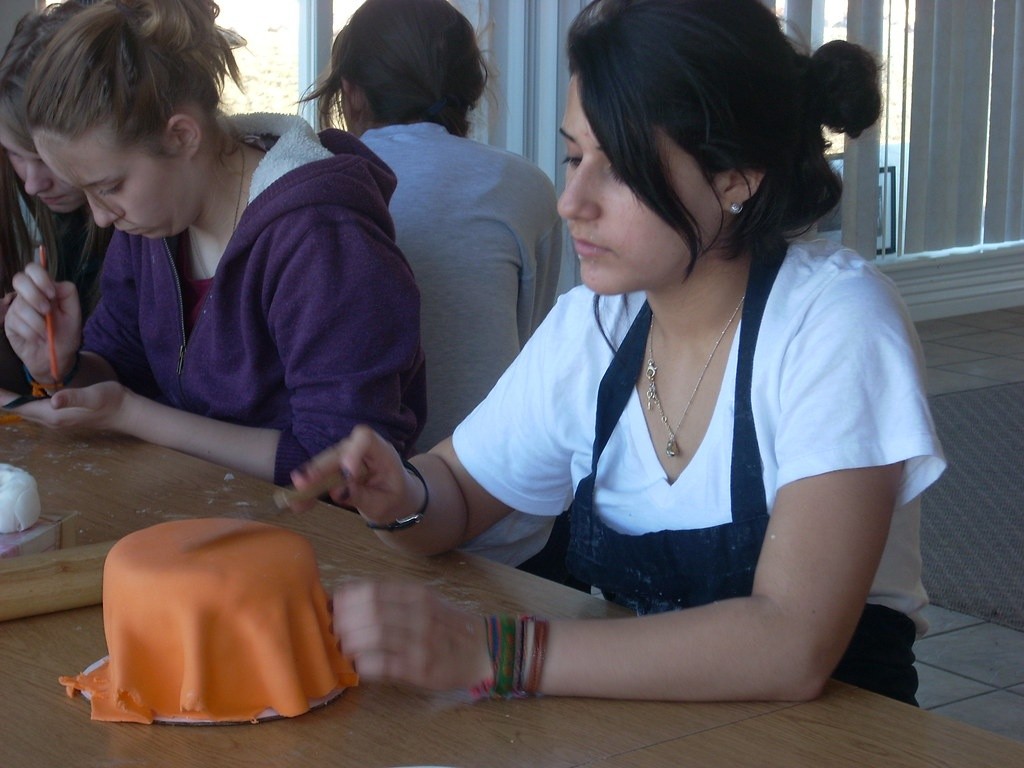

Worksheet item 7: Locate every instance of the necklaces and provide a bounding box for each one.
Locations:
[187,139,246,279]
[646,294,746,459]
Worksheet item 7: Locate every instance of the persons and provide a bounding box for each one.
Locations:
[293,0,564,575]
[286,0,946,708]
[0,0,430,512]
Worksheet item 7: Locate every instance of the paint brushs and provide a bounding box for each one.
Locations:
[39,243,63,389]
[263,468,371,512]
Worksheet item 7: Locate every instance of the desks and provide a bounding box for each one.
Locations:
[0,419,1024,767]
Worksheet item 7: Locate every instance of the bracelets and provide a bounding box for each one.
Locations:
[366,457,430,532]
[462,610,550,701]
[24,352,82,391]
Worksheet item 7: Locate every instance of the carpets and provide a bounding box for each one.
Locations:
[918,380,1024,631]
